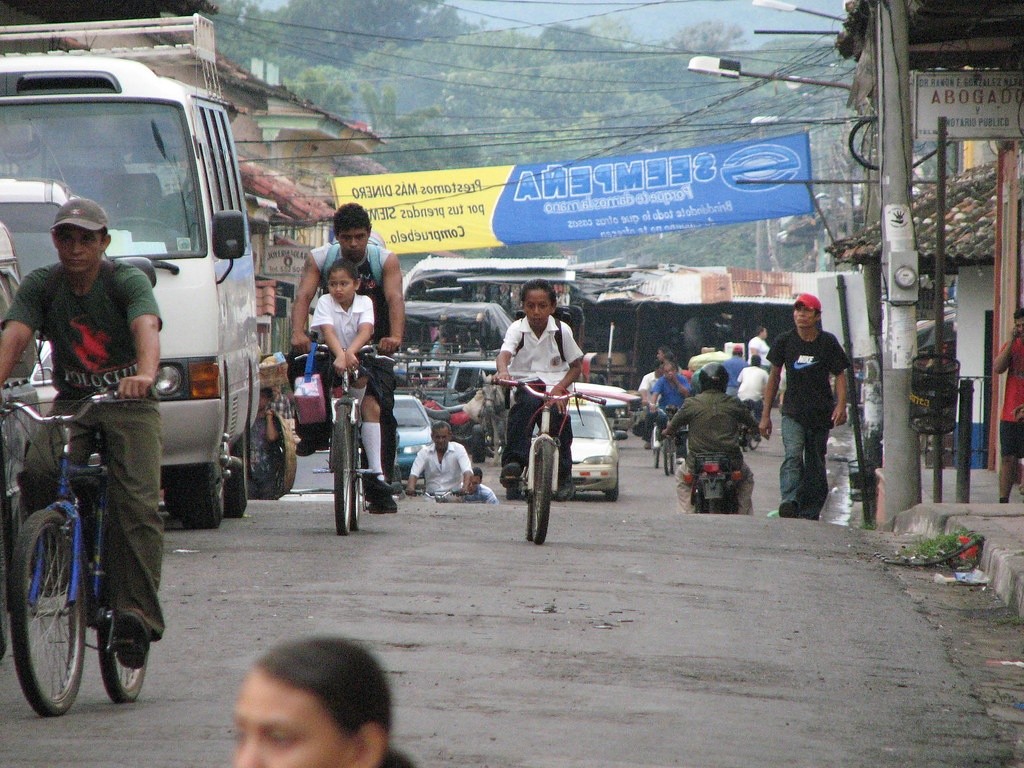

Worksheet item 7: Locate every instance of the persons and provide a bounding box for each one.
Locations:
[759,293,849,521]
[311,258,396,495]
[289,203,406,514]
[429,332,449,358]
[661,362,761,516]
[943,277,956,309]
[829,362,865,427]
[490,279,584,502]
[461,467,499,504]
[249,383,295,500]
[993,307,1024,503]
[638,326,787,464]
[405,421,474,502]
[0,198,166,668]
[229,634,418,768]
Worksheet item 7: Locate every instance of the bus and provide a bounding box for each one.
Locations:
[1,51,260,530]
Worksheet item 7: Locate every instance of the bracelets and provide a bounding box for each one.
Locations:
[265,413,274,416]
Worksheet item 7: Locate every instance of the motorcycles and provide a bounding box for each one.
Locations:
[670,430,758,514]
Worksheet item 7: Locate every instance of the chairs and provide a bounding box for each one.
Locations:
[115,171,167,218]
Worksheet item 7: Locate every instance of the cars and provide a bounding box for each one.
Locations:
[361,393,435,475]
[509,402,627,503]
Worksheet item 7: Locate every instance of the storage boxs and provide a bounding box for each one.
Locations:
[295,373,329,425]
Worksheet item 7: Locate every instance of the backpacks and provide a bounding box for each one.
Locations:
[515,306,571,363]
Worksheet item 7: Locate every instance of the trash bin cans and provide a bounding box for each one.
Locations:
[910,354,961,435]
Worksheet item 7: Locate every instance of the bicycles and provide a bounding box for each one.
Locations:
[0,385,150,717]
[491,378,608,547]
[645,403,763,475]
[296,347,396,535]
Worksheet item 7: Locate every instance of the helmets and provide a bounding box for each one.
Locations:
[698,363,729,392]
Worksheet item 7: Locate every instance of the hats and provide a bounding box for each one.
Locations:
[751,354,761,366]
[733,345,742,353]
[49,199,109,234]
[793,294,822,310]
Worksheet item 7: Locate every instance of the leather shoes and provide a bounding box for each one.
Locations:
[362,472,395,495]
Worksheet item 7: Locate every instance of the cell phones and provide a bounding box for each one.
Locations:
[1013,329,1018,338]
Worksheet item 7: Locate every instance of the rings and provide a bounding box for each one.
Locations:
[352,366,355,369]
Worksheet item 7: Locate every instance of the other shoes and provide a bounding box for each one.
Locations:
[378,496,398,513]
[554,479,576,501]
[676,457,686,464]
[644,442,650,448]
[295,433,331,456]
[112,609,150,669]
[778,500,800,519]
[499,461,522,488]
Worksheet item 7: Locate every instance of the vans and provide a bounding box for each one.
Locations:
[0,180,157,549]
